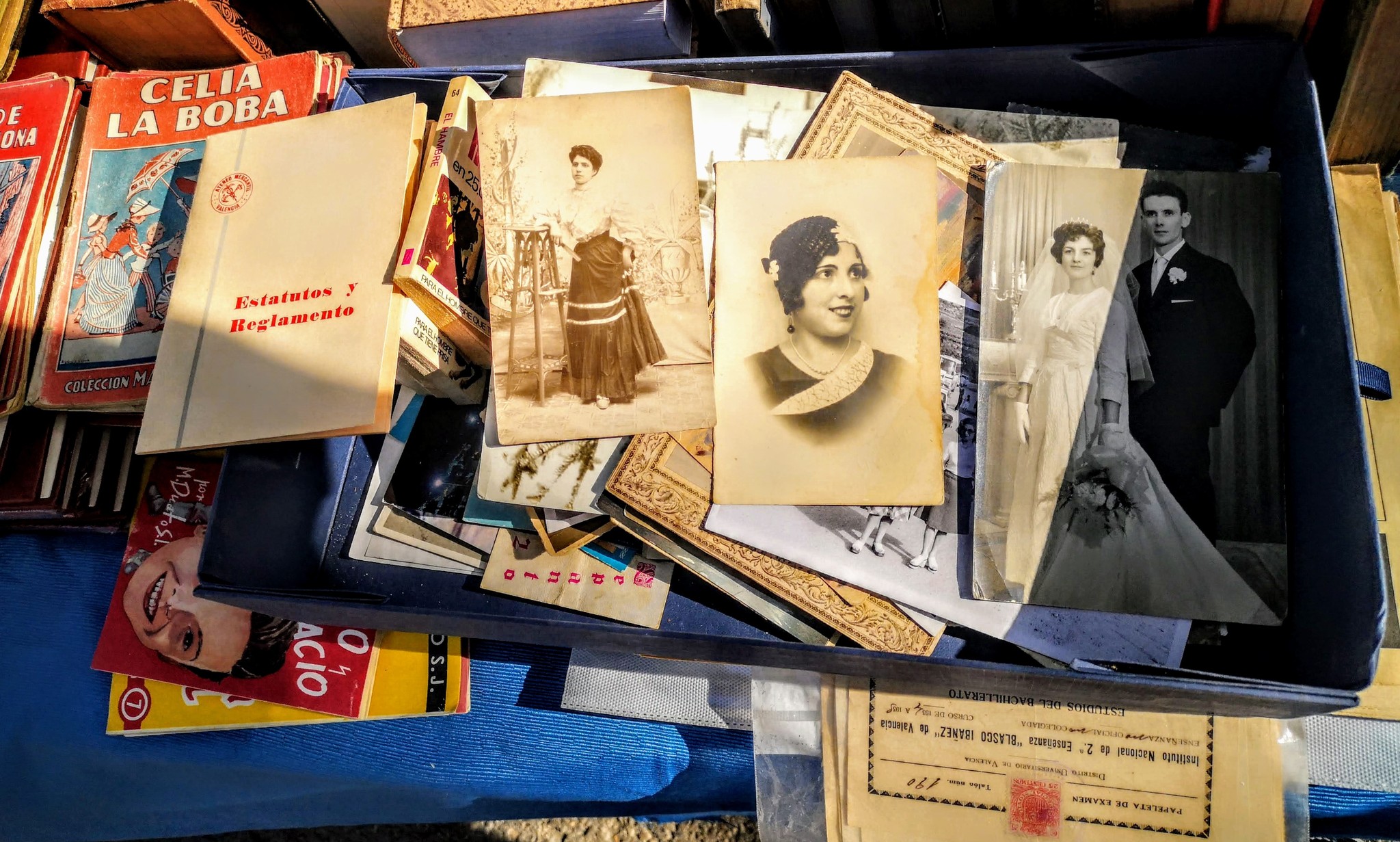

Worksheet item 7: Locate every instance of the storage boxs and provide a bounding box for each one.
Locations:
[191,29,1390,721]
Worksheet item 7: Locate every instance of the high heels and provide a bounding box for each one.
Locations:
[908,555,928,567]
[927,557,938,572]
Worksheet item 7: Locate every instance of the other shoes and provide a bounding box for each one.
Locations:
[872,541,885,556]
[597,394,610,408]
[850,539,864,554]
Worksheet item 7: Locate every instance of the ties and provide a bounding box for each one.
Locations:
[1153,257,1167,296]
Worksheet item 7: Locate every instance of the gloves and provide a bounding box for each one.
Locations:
[1097,423,1127,453]
[1013,402,1031,449]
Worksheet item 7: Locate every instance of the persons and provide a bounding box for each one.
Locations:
[1011,221,1153,597]
[744,214,914,440]
[1117,181,1259,549]
[536,145,668,410]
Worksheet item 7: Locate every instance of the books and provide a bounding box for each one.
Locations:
[0,0,1400,842]
